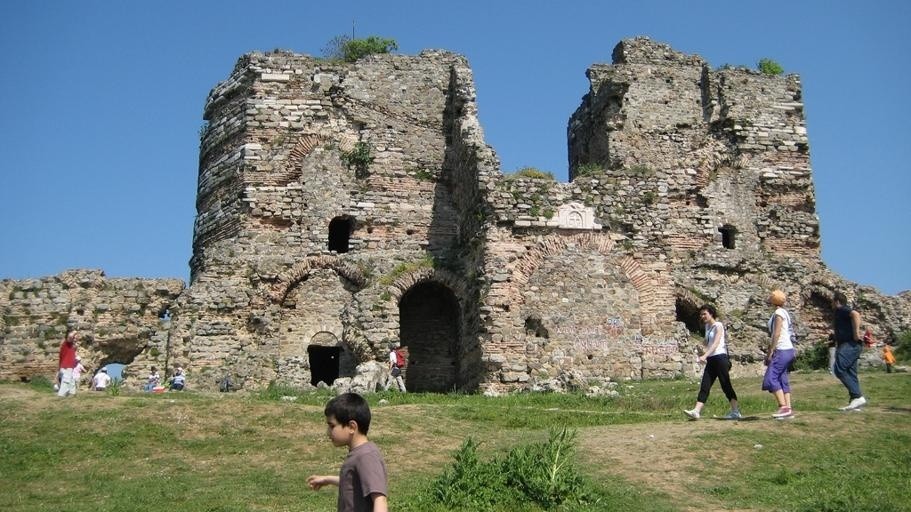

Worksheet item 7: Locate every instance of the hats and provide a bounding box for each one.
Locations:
[770,289,786,306]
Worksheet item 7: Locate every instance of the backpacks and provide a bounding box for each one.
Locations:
[395,349,405,367]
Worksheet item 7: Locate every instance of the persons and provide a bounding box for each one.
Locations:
[167,367,185,393]
[830,291,866,412]
[90,366,110,391]
[682,302,741,420]
[883,345,897,373]
[382,344,407,394]
[74,354,86,391]
[56,325,80,398]
[304,392,389,512]
[863,327,875,349]
[143,365,160,394]
[759,287,797,421]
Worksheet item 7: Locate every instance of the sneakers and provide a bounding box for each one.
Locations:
[772,407,795,420]
[838,396,866,412]
[683,409,701,419]
[720,409,741,420]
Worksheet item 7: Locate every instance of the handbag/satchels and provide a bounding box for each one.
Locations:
[728,361,732,370]
[391,368,400,377]
